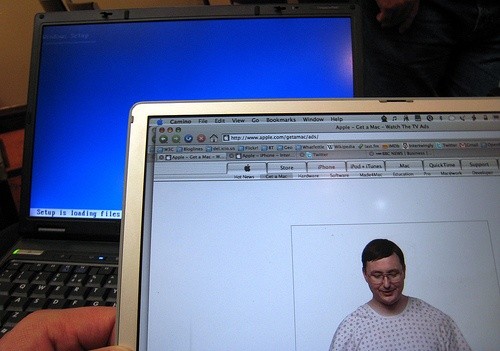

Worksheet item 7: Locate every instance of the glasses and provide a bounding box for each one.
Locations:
[366,268,403,284]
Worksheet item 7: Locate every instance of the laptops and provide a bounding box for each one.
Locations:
[0,4,364,334]
[115,97,500,351]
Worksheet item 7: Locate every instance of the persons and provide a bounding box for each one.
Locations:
[329,239,474,351]
